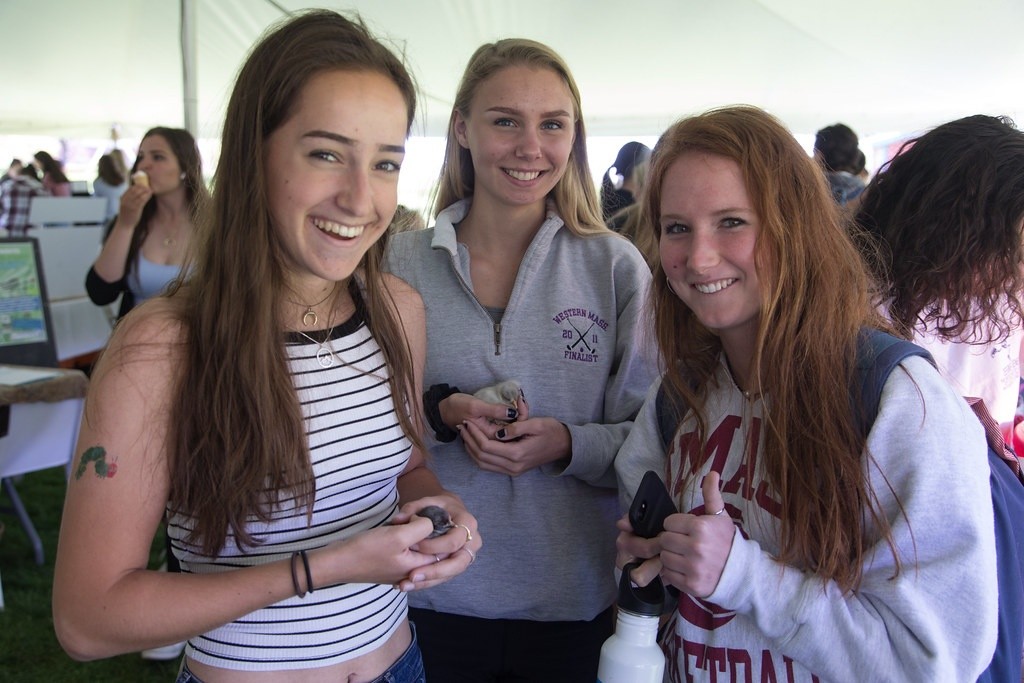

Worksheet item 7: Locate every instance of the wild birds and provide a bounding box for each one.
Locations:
[416,506,458,539]
[473,379,521,426]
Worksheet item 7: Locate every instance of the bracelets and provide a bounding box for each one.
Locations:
[291,550,306,599]
[301,549,316,594]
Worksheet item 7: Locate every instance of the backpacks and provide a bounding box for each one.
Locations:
[842,326,1024,683]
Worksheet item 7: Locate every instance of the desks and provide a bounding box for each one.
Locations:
[0,363,90,567]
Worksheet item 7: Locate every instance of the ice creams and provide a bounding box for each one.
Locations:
[132,170,149,187]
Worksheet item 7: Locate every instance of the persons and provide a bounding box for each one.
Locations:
[53,8,487,683]
[606,105,1001,683]
[87,124,214,326]
[0,122,871,247]
[847,110,1024,468]
[373,39,667,683]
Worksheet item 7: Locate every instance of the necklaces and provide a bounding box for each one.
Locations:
[159,216,183,248]
[283,282,338,327]
[741,388,764,398]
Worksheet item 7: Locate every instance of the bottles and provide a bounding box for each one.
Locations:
[597,560,666,683]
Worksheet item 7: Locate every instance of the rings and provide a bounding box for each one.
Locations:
[713,507,725,515]
[455,524,472,542]
[434,554,440,562]
[464,547,475,565]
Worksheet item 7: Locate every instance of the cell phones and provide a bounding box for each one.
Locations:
[630,471,681,597]
